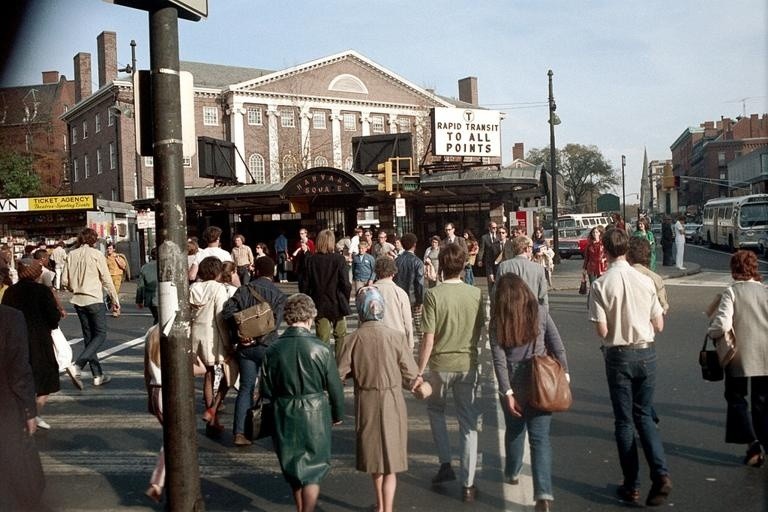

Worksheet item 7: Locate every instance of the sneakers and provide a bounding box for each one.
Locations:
[144,484,164,506]
[279,279,289,283]
[232,433,253,445]
[93,371,113,386]
[64,361,84,390]
[35,416,51,431]
[17,257,43,281]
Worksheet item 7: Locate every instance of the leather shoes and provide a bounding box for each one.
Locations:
[646,475,673,507]
[616,484,640,504]
[533,499,551,512]
[462,485,478,502]
[504,478,519,484]
[431,467,456,484]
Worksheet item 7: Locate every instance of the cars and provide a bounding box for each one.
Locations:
[540,227,593,260]
[647,222,704,242]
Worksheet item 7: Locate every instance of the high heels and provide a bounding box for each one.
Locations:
[201,409,226,441]
[743,440,768,469]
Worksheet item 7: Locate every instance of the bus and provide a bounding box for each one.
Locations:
[556,211,617,231]
[702,193,767,254]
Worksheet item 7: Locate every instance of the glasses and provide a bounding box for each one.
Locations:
[498,232,507,234]
[256,247,262,249]
[491,227,498,229]
[445,228,453,231]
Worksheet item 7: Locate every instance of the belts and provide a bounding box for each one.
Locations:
[606,340,656,352]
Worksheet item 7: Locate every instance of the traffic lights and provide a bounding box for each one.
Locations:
[674,176,680,188]
[377,162,391,191]
[402,175,421,193]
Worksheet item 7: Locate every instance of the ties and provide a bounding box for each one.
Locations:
[492,232,495,242]
[494,242,504,265]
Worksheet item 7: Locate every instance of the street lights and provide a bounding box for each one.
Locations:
[547,67,564,263]
[620,154,628,229]
[111,39,149,277]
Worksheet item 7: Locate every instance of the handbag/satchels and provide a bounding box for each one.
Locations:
[527,335,574,413]
[283,260,294,272]
[698,333,725,382]
[579,273,587,295]
[231,283,276,340]
